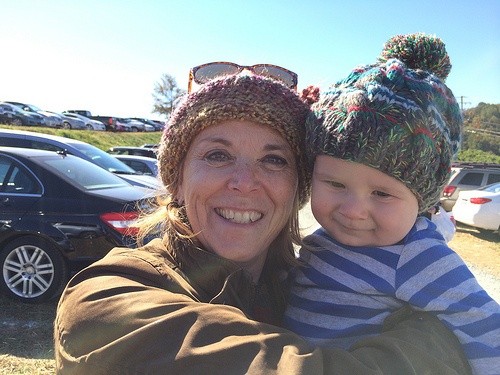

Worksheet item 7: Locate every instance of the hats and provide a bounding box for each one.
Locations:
[157,72,320,211]
[303,34,463,215]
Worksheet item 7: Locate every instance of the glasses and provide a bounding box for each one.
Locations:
[188,61,299,97]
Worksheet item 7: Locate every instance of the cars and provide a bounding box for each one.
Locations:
[0,101,166,133]
[451,180,500,240]
[0,129,170,194]
[91,144,161,182]
[0,146,169,305]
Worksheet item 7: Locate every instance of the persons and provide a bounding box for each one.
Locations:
[54,75,471,375]
[281,35,500,375]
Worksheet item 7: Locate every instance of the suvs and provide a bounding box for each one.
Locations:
[439,163,500,212]
